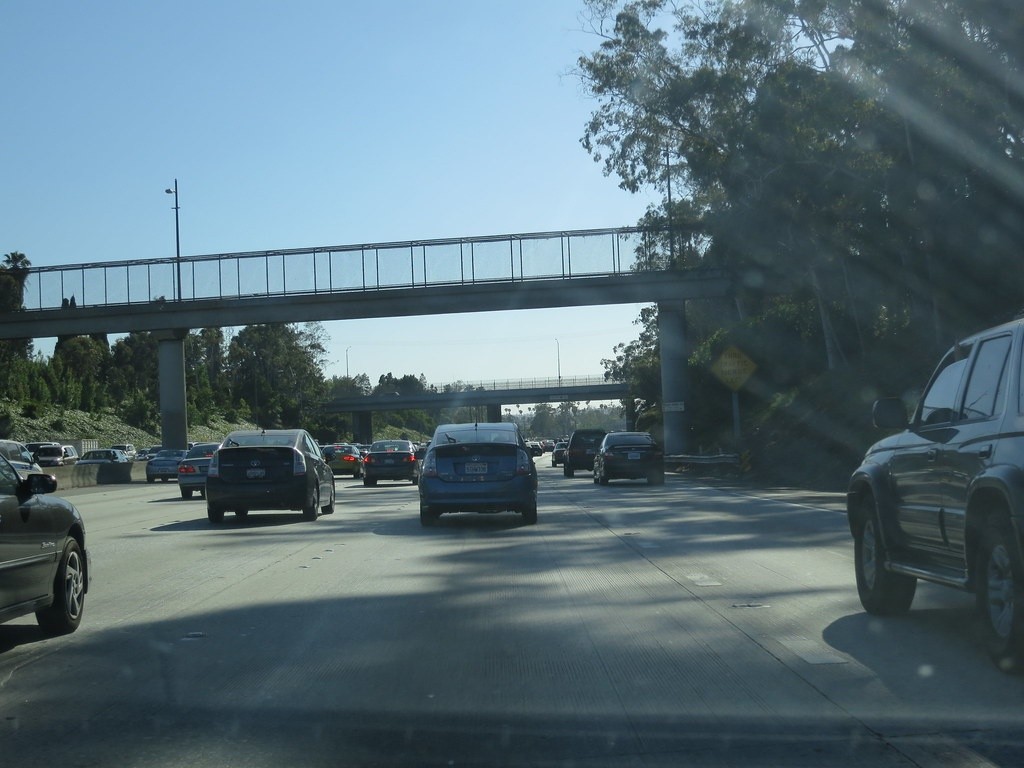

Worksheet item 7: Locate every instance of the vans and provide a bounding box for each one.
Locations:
[22,442,60,463]
[32,445,79,466]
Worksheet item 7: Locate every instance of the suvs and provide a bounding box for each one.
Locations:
[848,315,1023,677]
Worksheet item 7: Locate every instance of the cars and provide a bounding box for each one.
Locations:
[315,438,433,459]
[362,440,421,486]
[187,442,198,449]
[562,429,608,478]
[552,440,569,467]
[205,429,335,524]
[75,449,130,464]
[409,422,539,526]
[0,451,92,635]
[591,433,667,488]
[523,438,565,457]
[177,442,222,499]
[136,445,161,460]
[145,448,188,481]
[319,444,364,479]
[0,440,46,482]
[111,444,137,460]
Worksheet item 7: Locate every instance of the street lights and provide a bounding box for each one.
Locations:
[164,187,183,303]
